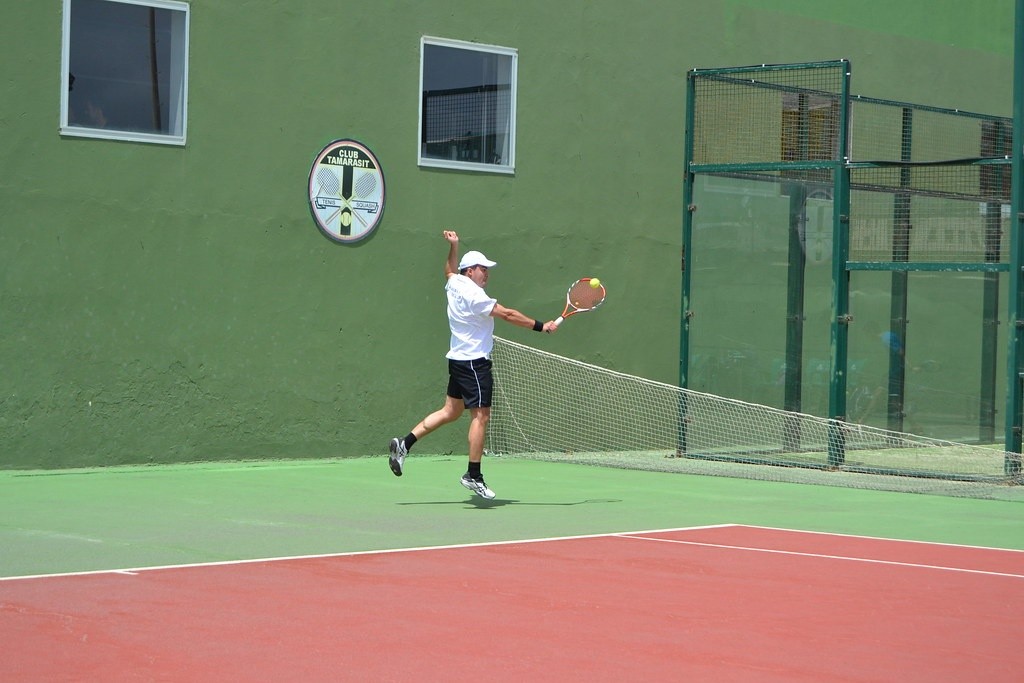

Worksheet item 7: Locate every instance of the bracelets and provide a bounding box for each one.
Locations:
[532,319,543,332]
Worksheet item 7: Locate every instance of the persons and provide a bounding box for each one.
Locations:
[389,230,558,499]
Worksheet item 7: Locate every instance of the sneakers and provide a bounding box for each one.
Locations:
[388,437,409,477]
[460,471,495,500]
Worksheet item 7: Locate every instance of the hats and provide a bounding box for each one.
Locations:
[458,250,497,270]
